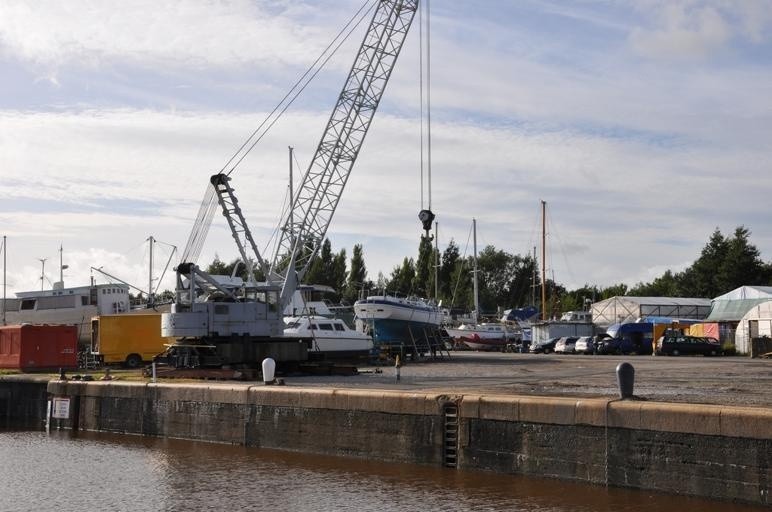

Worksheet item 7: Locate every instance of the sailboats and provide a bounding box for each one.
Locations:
[444,200,560,351]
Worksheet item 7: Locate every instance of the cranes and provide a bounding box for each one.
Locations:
[142,0,434,377]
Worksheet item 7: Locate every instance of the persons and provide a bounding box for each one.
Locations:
[59,368,68,380]
[100,368,112,380]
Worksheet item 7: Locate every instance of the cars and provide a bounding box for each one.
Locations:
[529,335,635,354]
[657,336,721,356]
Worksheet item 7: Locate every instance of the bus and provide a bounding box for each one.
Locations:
[606,322,654,354]
[560,312,592,324]
